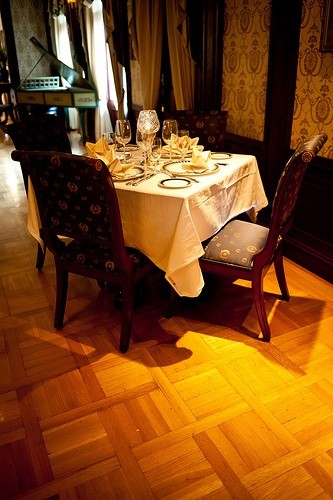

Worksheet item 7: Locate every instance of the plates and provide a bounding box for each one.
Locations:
[210,152,232,159]
[112,165,145,180]
[162,160,219,176]
[116,152,131,160]
[157,178,192,188]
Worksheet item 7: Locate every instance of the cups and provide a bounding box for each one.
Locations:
[137,110,160,134]
[104,132,115,149]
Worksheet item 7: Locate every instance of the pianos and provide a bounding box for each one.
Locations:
[14,36,98,147]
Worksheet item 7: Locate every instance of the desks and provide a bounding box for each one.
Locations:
[24,150,269,299]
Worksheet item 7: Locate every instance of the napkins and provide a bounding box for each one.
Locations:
[190,147,211,174]
[162,133,204,154]
[85,136,117,158]
[94,151,136,178]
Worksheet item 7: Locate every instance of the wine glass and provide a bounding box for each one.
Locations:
[177,129,189,161]
[162,120,178,164]
[115,120,132,164]
[137,132,161,177]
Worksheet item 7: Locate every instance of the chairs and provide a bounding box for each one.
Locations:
[2,116,73,269]
[165,132,327,341]
[159,110,229,152]
[11,149,158,353]
